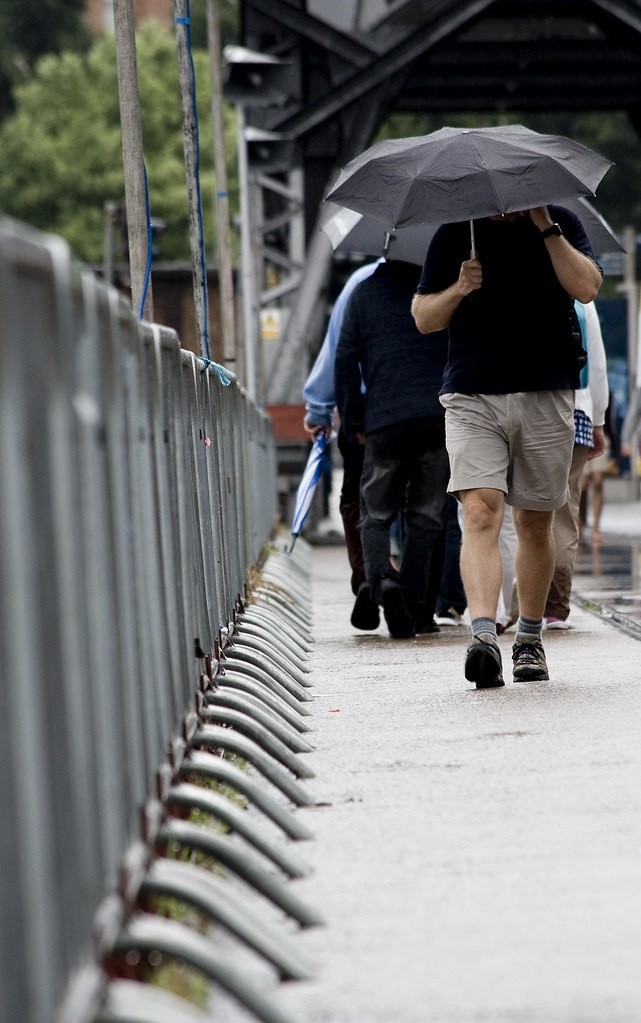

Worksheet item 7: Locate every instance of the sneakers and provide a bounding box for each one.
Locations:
[512,631,549,681]
[464,632,505,688]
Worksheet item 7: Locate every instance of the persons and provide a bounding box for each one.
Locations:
[301,255,471,637]
[409,203,604,690]
[493,294,610,636]
[620,387,641,457]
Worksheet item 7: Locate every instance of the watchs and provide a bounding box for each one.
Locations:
[541,222,564,239]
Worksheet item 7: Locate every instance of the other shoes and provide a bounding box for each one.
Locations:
[378,575,416,639]
[351,580,381,630]
[545,616,570,630]
[433,610,460,626]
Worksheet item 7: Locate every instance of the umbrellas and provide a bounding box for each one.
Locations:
[322,122,616,261]
[317,194,626,267]
[286,431,330,554]
